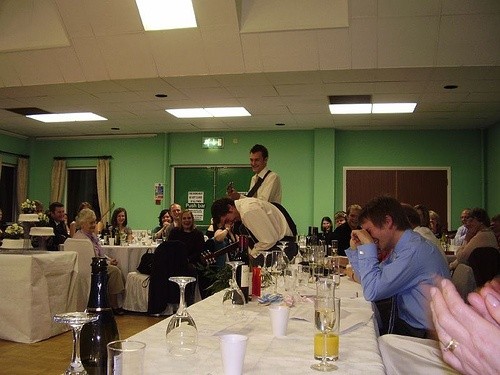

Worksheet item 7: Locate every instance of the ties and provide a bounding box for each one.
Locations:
[386,252,398,333]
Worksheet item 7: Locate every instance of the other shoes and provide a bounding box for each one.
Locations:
[112,308,124,315]
[150,311,160,317]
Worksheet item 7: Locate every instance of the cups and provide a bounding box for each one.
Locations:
[314,297,340,360]
[109,238,114,245]
[107,340,146,375]
[284,269,297,292]
[220,334,248,375]
[329,276,339,288]
[438,238,467,251]
[100,239,104,245]
[131,228,167,246]
[298,266,310,286]
[267,306,290,337]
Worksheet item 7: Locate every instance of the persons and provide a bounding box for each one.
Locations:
[226,144,282,204]
[29,196,500,375]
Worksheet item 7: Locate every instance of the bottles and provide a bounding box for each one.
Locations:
[440,231,448,252]
[99,221,115,245]
[80,256,123,375]
[115,227,120,245]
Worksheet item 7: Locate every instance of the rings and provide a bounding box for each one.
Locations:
[443,338,459,353]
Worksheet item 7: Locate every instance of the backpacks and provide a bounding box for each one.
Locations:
[137,247,154,274]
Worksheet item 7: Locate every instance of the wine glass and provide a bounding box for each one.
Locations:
[257,235,339,372]
[166,276,198,357]
[222,261,249,323]
[52,312,99,375]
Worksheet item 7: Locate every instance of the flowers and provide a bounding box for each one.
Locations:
[21,198,37,209]
[39,212,49,224]
[4,223,25,235]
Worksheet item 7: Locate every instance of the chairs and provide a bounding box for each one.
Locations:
[450,264,477,301]
[122,239,187,318]
[63,238,110,310]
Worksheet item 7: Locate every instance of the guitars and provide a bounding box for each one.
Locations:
[199,222,254,265]
[226,181,235,201]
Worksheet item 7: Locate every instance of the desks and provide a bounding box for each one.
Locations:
[59,242,162,308]
[0,250,78,343]
[122,273,389,374]
